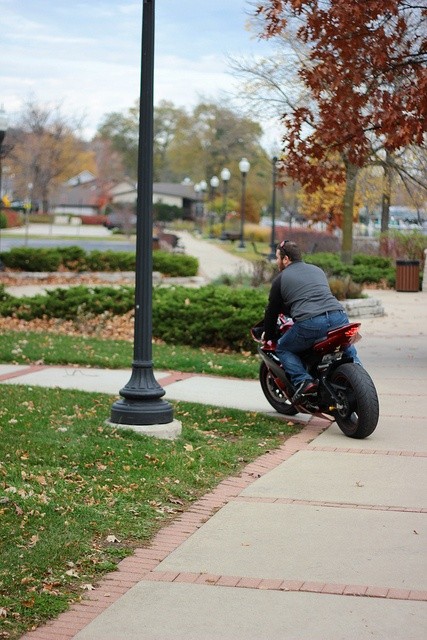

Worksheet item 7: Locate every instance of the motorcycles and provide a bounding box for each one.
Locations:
[251,313,379,438]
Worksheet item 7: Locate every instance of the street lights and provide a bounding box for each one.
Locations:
[194,184,201,231]
[221,168,231,240]
[209,176,219,238]
[239,158,250,247]
[269,140,279,259]
[199,180,207,233]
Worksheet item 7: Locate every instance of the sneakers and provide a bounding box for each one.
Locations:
[292,379,317,405]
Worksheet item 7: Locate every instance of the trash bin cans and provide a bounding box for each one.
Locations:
[395,260,420,292]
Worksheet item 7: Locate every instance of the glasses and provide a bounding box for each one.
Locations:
[280,240,288,256]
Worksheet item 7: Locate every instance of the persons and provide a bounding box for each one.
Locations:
[262,240,349,406]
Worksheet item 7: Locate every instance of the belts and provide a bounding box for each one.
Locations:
[312,310,338,318]
